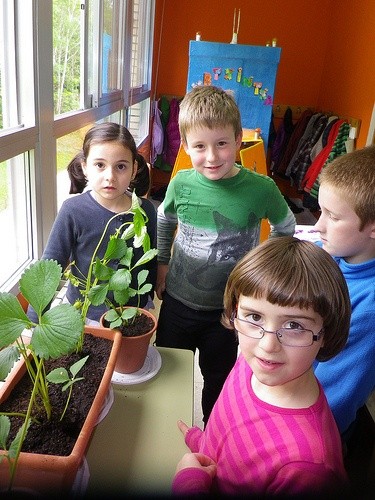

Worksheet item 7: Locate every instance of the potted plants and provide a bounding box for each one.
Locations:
[85,187,160,374]
[0,259,124,500]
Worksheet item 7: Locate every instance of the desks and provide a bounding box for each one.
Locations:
[86,347,195,500]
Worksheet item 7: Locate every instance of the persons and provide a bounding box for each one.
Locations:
[171,236,351,494]
[299,146,375,455]
[155,86,297,430]
[27,122,157,328]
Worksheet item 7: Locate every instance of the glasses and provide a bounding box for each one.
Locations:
[230,309,324,347]
